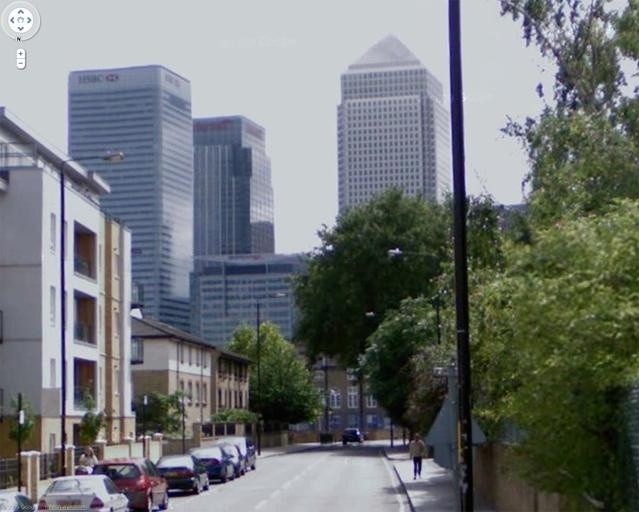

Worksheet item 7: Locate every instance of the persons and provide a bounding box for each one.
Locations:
[408,430,426,481]
[78,445,99,466]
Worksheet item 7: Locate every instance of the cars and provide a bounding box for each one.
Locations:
[343,428,364,444]
[38,437,255,512]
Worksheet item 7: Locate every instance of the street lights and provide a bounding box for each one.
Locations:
[61,152,125,476]
[387,248,441,348]
[143,392,148,459]
[319,349,331,432]
[256,293,286,455]
